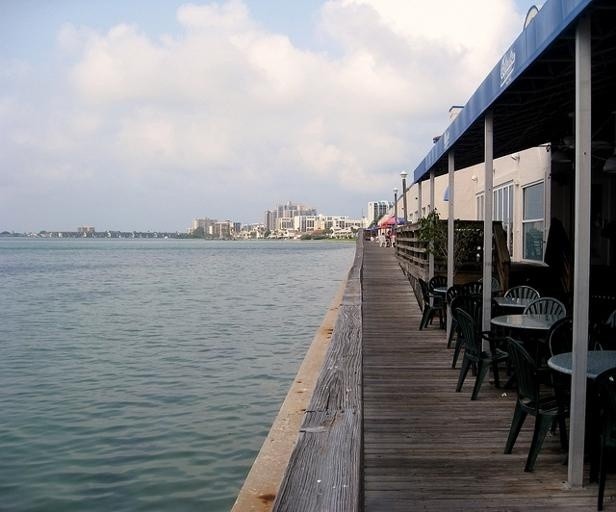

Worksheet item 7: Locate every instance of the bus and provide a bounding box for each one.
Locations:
[364,227,377,241]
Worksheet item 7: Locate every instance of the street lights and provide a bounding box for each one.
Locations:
[399,169,408,224]
[392,187,399,228]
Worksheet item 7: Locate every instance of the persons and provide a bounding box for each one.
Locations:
[382,230,391,247]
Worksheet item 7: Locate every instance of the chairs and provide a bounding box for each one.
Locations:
[370,235,396,248]
[503,318,615,511]
[416,274,613,400]
